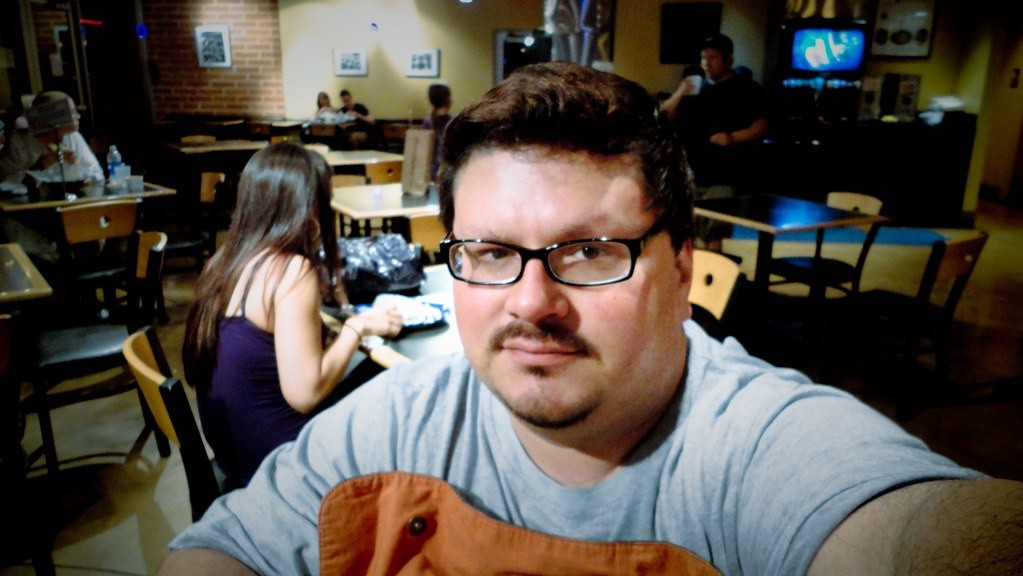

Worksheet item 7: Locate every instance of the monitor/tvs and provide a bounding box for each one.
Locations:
[776,17,867,81]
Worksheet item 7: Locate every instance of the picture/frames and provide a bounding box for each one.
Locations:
[405,49,442,78]
[334,47,367,77]
[194,26,233,68]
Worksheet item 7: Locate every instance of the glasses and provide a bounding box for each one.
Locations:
[438,208,677,286]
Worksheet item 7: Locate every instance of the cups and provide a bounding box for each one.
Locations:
[109,165,144,194]
[687,74,701,96]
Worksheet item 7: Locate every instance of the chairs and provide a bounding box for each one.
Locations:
[0,117,990,576]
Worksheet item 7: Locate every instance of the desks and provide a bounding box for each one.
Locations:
[0,242,52,304]
[692,195,890,298]
[0,177,177,310]
[330,182,442,245]
[165,140,269,203]
[320,150,404,239]
[320,264,459,377]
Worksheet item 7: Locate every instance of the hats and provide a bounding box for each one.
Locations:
[699,34,733,56]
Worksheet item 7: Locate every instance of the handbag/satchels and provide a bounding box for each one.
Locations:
[318,232,424,304]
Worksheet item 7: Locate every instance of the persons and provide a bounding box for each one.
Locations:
[0,92,105,193]
[338,90,374,125]
[424,84,453,180]
[658,34,770,183]
[183,140,404,488]
[315,92,334,116]
[159,66,1023,576]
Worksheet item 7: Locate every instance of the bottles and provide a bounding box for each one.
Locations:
[107,146,121,178]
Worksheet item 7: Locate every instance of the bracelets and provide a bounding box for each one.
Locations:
[726,132,732,142]
[343,322,361,344]
